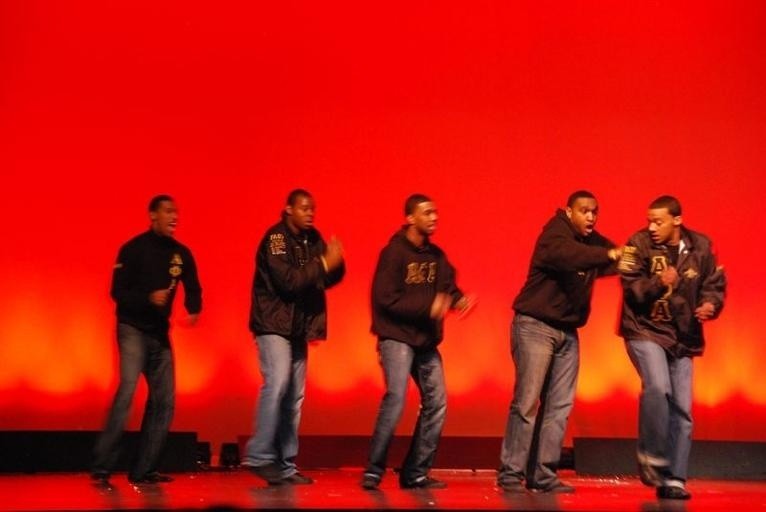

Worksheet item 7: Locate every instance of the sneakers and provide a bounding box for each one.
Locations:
[498,481,573,500]
[128,472,173,482]
[249,463,313,485]
[92,466,109,479]
[639,464,690,500]
[400,474,447,489]
[363,476,381,490]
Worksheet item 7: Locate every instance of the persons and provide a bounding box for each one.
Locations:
[614,193,727,501]
[238,188,347,486]
[495,188,623,493]
[359,192,471,490]
[86,192,202,484]
